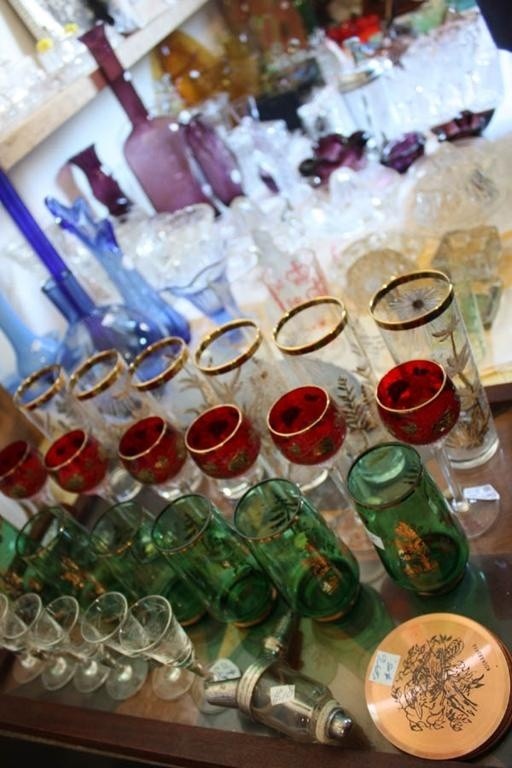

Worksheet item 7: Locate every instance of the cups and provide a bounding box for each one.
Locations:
[66,349,203,503]
[193,318,332,493]
[13,363,144,503]
[370,269,500,469]
[4,497,281,629]
[124,336,264,500]
[271,294,391,461]
[346,441,468,597]
[261,246,330,313]
[224,474,373,627]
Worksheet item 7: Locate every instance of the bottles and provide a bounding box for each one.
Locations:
[0,22,222,392]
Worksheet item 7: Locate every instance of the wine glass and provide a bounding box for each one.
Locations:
[118,594,243,715]
[0,428,124,512]
[373,357,502,539]
[80,589,198,701]
[184,402,264,496]
[265,384,377,553]
[35,595,149,702]
[116,414,194,501]
[0,592,79,691]
[0,592,47,684]
